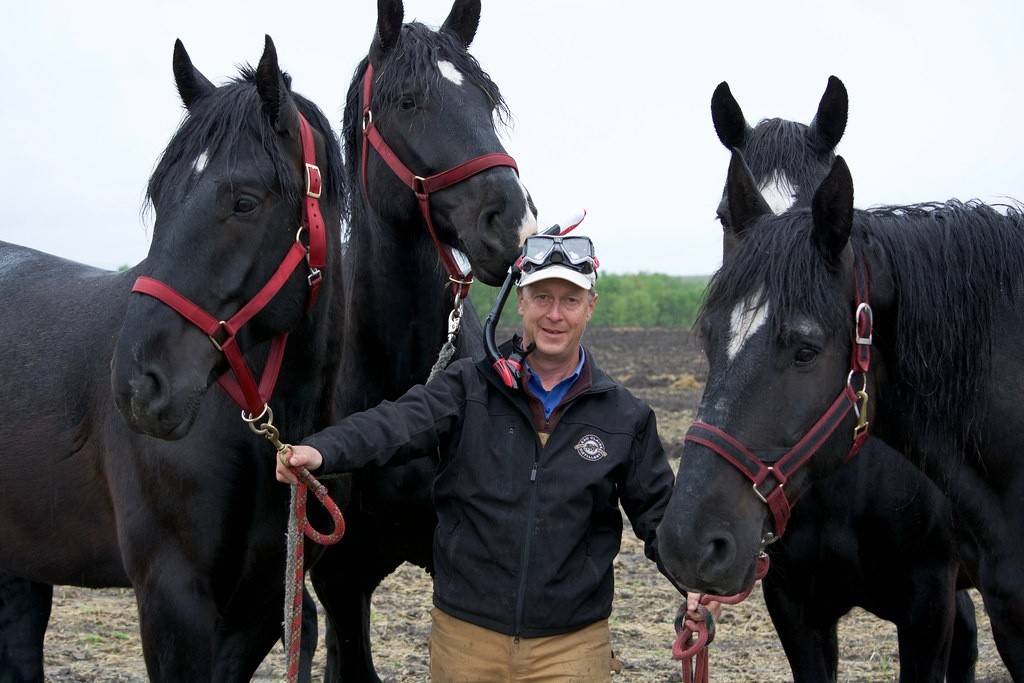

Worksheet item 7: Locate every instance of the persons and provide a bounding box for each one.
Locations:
[276,231,723,683]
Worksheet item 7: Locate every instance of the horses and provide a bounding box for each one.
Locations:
[652,76,1024,682]
[0,1,538,683]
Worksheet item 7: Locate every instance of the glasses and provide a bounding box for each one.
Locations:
[512,234,600,284]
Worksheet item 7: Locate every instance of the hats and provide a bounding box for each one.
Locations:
[514,250,596,290]
